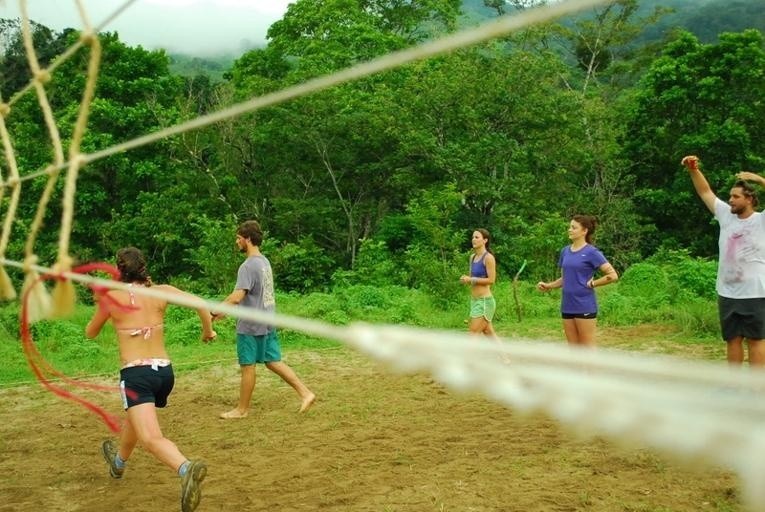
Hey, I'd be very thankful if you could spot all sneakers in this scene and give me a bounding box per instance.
[179,461,207,511]
[103,439,124,478]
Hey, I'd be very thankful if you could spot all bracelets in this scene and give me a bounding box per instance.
[472,277,478,284]
[210,312,218,317]
[590,277,595,288]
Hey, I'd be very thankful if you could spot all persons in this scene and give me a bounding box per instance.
[212,220,315,419]
[681,155,765,393]
[537,214,618,374]
[85,247,216,512]
[460,228,508,369]
